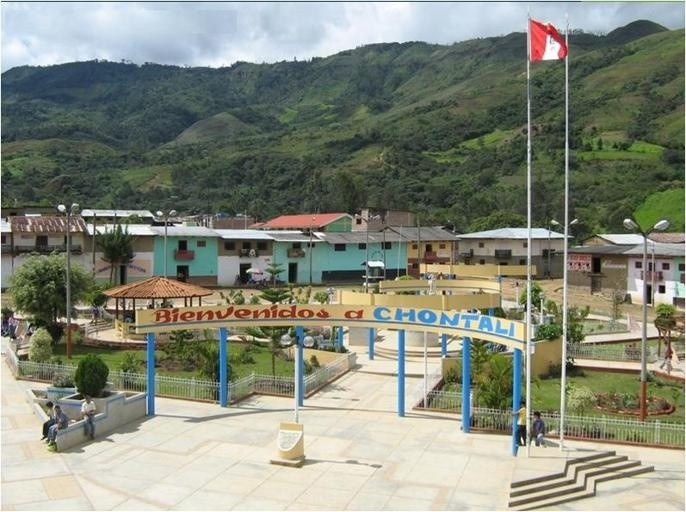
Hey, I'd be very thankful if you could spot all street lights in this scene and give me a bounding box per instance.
[355,211,385,293]
[551,217,578,287]
[57,200,81,359]
[308,215,317,286]
[623,217,671,422]
[155,208,179,278]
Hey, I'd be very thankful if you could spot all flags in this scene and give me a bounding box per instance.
[528,15,567,62]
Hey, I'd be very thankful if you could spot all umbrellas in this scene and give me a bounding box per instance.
[246,267,264,275]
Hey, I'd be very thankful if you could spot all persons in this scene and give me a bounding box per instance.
[41,401,55,440]
[26,323,33,336]
[8,314,15,338]
[89,305,98,326]
[533,411,545,447]
[81,396,96,440]
[511,401,526,445]
[45,406,68,445]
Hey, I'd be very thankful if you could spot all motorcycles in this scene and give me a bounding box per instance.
[254,277,270,290]
